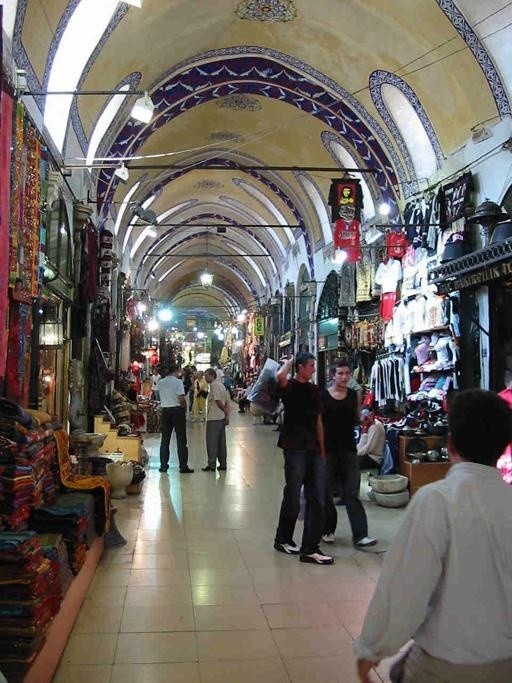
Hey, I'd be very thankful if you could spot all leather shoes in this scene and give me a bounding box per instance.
[217,466,226,470]
[202,465,215,472]
[180,467,194,472]
[159,467,167,472]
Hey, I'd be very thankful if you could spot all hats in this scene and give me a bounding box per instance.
[413,332,451,367]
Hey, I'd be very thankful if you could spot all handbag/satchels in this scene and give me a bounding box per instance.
[201,390,208,399]
[246,357,288,412]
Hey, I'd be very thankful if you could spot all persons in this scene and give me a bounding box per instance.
[151,364,252,472]
[274,352,387,565]
[354,388,512,682]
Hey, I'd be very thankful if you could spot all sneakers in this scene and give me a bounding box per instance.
[189,409,205,422]
[300,550,334,564]
[274,540,301,553]
[322,531,335,541]
[356,536,377,546]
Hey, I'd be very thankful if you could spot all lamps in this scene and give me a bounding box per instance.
[22,91,154,124]
[61,163,129,181]
[364,224,439,244]
[335,246,404,263]
[200,223,214,288]
